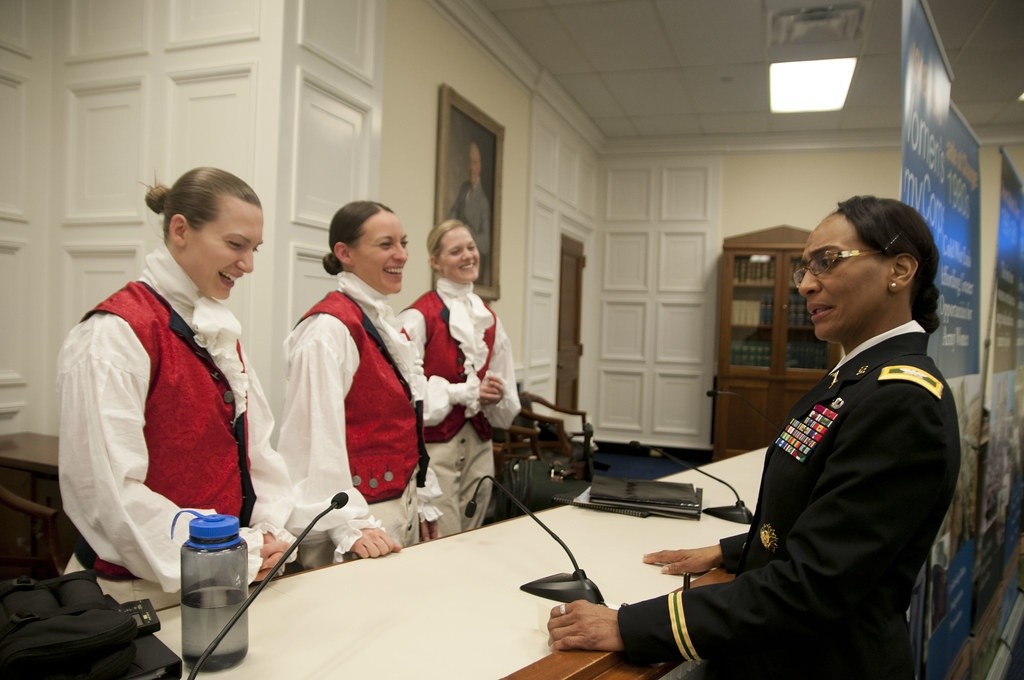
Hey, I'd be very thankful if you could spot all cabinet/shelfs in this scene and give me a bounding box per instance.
[708,225,842,458]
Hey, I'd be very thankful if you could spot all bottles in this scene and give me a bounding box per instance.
[171,511,248,673]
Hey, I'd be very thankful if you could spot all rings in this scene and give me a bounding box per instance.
[557,602,569,615]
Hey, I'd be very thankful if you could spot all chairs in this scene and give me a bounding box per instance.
[487,394,587,487]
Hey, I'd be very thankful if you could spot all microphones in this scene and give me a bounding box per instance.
[464,474,604,606]
[629,441,754,525]
[186,492,349,680]
[706,390,781,429]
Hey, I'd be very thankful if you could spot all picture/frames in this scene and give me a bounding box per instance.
[427,83,507,303]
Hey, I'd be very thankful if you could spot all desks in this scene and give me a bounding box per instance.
[152,447,770,680]
[0,433,58,570]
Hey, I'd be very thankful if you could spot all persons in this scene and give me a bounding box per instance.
[58,166,302,613]
[276,200,441,566]
[547,194,962,680]
[396,220,522,540]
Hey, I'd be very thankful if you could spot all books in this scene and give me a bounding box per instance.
[728,258,829,369]
[552,476,704,521]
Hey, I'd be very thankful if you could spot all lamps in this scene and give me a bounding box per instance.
[763,40,859,117]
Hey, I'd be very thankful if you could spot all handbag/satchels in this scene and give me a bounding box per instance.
[0,570,136,680]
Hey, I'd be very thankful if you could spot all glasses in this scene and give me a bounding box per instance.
[794,250,881,288]
